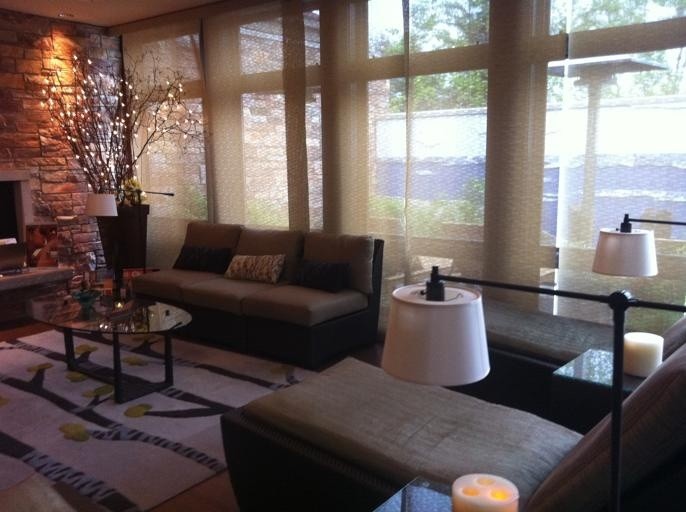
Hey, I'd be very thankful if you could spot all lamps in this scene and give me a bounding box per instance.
[87,194,118,216]
[381,266,686,512]
[592,214,686,277]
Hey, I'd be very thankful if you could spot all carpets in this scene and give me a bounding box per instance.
[0,319,319,512]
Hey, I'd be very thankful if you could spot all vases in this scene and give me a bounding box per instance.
[96,204,150,290]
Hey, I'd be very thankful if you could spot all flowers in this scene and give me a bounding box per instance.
[115,178,147,206]
[44,53,213,192]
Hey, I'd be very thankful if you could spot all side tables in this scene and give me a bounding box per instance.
[553,346,645,393]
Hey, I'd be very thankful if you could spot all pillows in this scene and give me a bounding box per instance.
[282,262,349,292]
[173,245,231,272]
[225,254,284,284]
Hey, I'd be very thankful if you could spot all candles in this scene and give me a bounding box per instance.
[453,472,520,512]
[622,331,663,379]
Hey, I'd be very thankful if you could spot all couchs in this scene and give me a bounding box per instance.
[131,219,384,369]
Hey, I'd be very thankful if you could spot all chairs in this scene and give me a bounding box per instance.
[222,341,686,512]
[482,298,686,373]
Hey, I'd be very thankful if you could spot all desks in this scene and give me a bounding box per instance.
[0,267,74,299]
